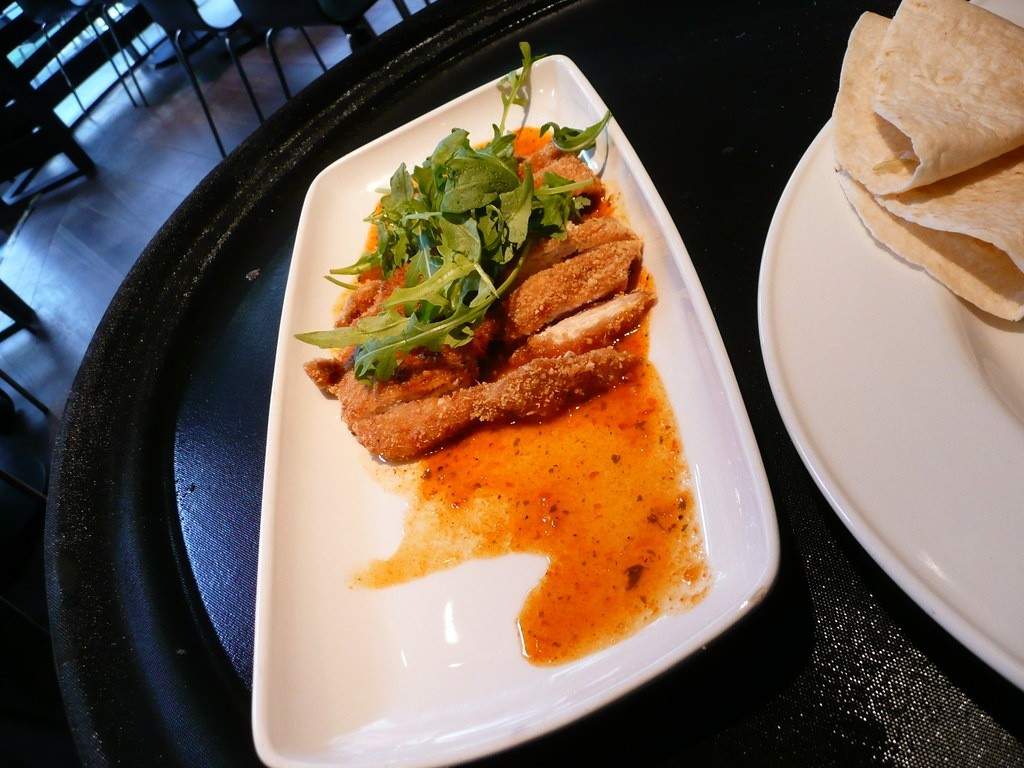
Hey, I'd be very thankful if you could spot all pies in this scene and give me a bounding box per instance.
[831,0,1024,323]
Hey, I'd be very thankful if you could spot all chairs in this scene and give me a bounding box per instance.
[0,0,435,243]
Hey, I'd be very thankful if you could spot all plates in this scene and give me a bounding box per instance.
[248,55,780,768]
[755,110,1024,689]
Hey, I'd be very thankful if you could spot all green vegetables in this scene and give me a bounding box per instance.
[291,42,612,388]
